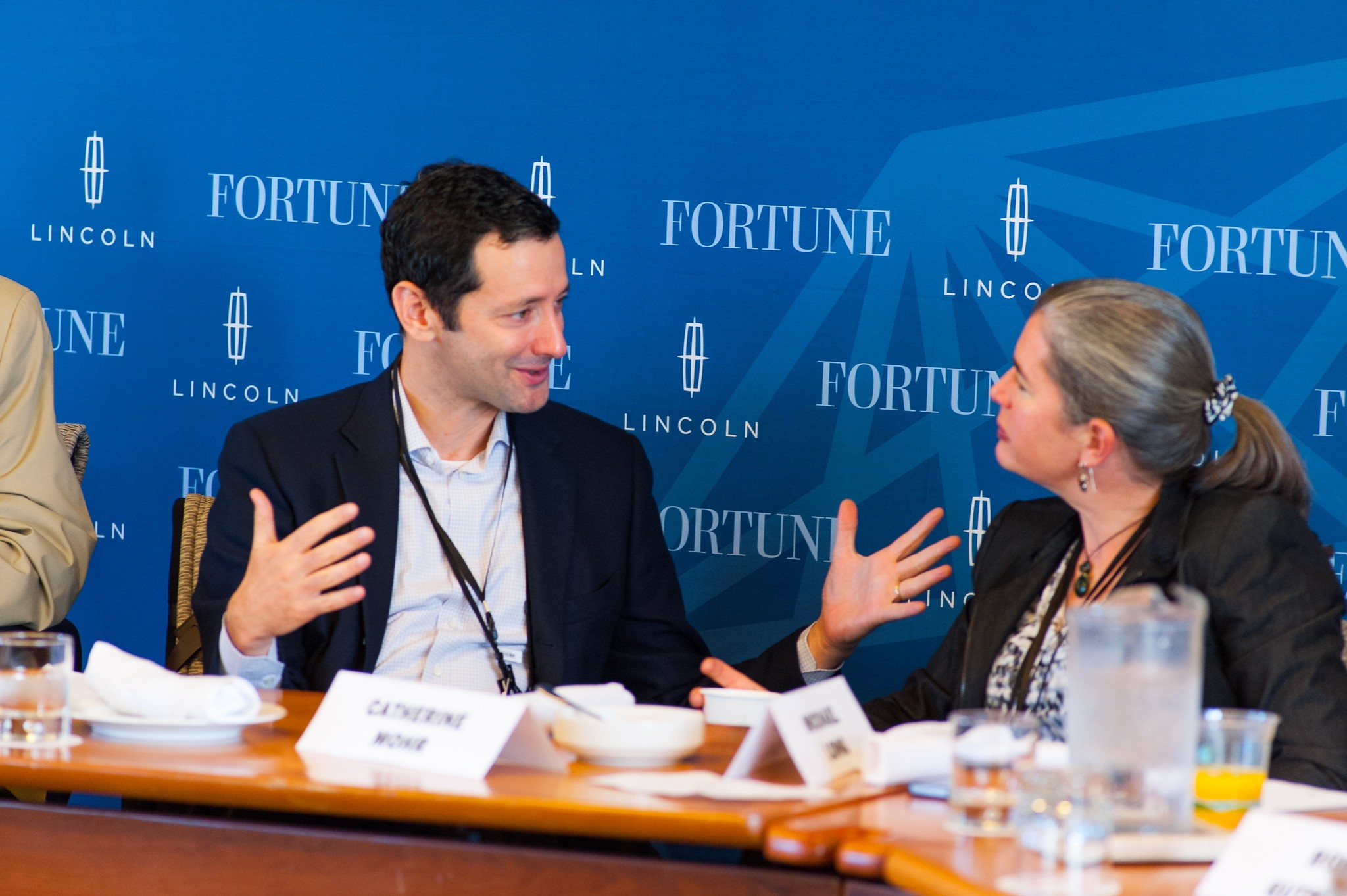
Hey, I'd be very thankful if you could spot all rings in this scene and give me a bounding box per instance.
[895,583,899,595]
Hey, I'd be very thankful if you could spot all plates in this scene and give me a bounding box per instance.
[550,704,706,766]
[71,704,290,747]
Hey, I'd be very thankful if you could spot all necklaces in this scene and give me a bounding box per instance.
[1075,516,1147,597]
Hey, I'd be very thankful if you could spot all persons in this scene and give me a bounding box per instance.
[0,275,96,632]
[190,158,960,709]
[690,279,1347,788]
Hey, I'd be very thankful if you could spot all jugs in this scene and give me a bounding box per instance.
[1065,595,1207,838]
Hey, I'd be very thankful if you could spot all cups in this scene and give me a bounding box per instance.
[1196,709,1283,831]
[0,632,75,752]
[948,708,1037,838]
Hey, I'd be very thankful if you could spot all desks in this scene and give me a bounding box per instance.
[1,668,1208,896]
